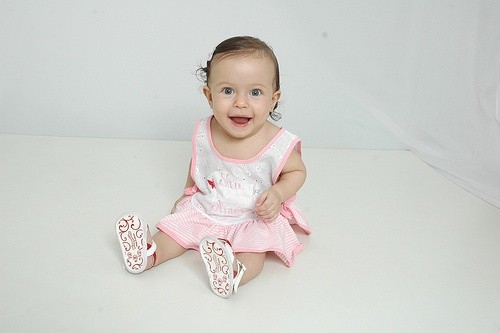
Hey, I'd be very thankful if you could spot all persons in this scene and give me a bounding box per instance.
[114,37,312,298]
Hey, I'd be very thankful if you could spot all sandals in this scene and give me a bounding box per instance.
[200,237,246,298]
[115,215,157,273]
